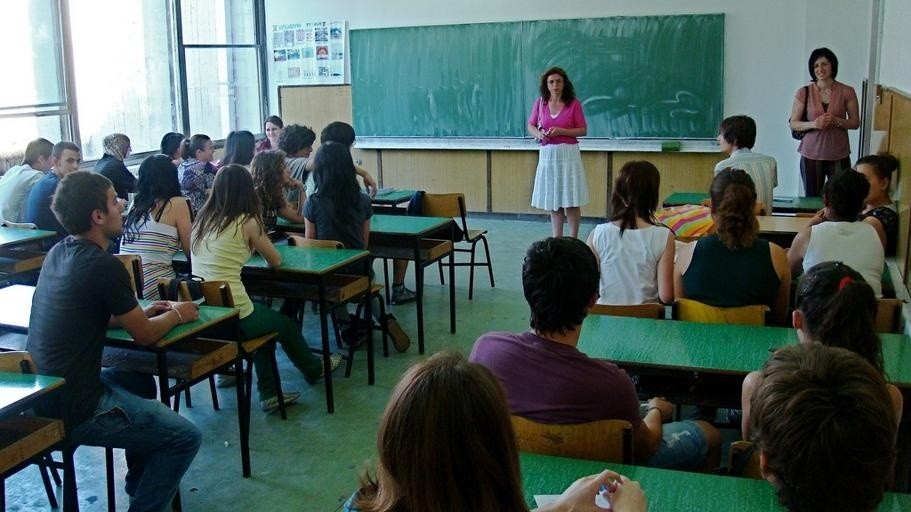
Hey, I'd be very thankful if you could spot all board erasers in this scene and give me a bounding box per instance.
[659,144,681,154]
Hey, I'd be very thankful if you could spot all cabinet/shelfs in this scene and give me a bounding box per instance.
[489,150,611,220]
[380,148,490,216]
[278,84,381,189]
[608,152,730,213]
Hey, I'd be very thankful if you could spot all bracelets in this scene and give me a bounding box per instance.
[835,116,845,128]
[645,406,667,418]
[170,305,184,327]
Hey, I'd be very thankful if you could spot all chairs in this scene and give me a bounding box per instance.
[701,198,765,214]
[672,298,769,325]
[413,191,495,300]
[874,298,904,334]
[2,350,110,512]
[158,276,288,442]
[2,220,39,230]
[289,234,389,377]
[112,254,144,302]
[588,302,665,320]
[507,412,634,467]
[735,441,777,481]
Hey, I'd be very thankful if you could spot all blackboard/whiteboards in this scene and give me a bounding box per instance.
[348,13,725,141]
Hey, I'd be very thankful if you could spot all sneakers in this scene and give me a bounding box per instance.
[380,313,410,353]
[391,288,417,305]
[305,353,342,383]
[216,366,247,388]
[341,324,369,347]
[261,391,301,411]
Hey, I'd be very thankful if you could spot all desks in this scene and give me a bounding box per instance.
[0,367,79,512]
[0,285,251,512]
[517,450,911,512]
[277,215,457,356]
[663,192,823,214]
[176,244,375,415]
[362,186,421,201]
[575,313,911,410]
[0,227,58,284]
[719,215,811,249]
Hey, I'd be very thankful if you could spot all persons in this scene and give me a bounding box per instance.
[188,164,342,413]
[160,132,188,174]
[220,131,253,171]
[178,135,220,216]
[248,150,304,234]
[584,160,676,402]
[751,342,896,512]
[528,67,586,237]
[116,153,245,387]
[305,143,411,352]
[24,170,203,511]
[24,144,121,253]
[728,263,904,497]
[305,122,420,306]
[258,116,285,153]
[0,138,53,228]
[464,236,722,472]
[715,116,779,215]
[791,48,860,195]
[780,175,886,322]
[676,167,790,426]
[277,125,319,208]
[846,156,900,257]
[344,352,649,511]
[94,136,140,201]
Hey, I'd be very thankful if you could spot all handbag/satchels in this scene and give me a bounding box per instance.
[535,96,544,144]
[788,86,809,141]
